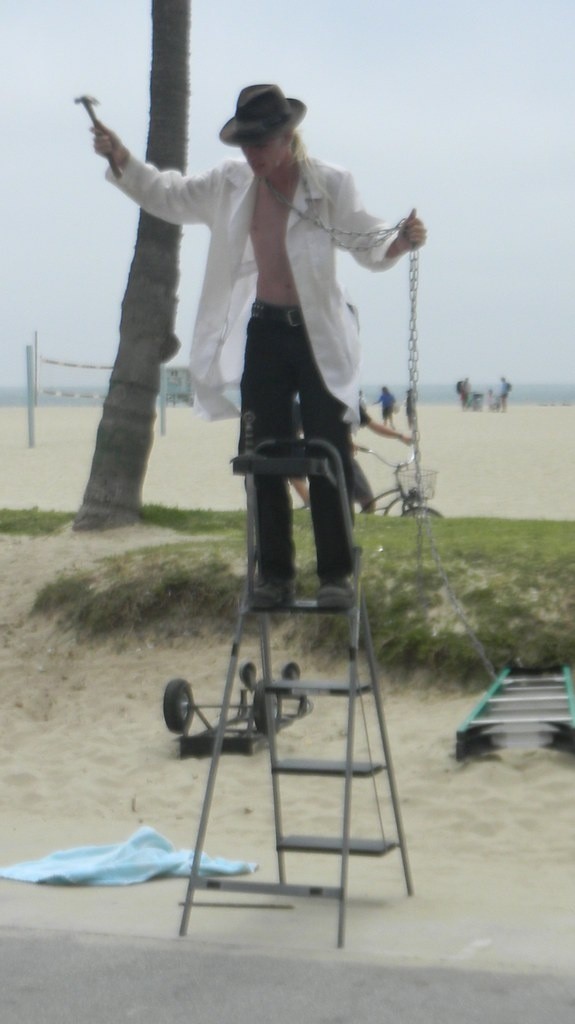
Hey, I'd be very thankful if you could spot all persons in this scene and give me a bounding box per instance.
[91,84,426,610]
[282,378,512,516]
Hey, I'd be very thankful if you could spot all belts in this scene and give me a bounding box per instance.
[251,303,302,326]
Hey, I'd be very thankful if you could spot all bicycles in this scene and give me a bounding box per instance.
[289,441,448,524]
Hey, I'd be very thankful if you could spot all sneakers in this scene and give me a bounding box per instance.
[249,579,296,608]
[318,575,354,607]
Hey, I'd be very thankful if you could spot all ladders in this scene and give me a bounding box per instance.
[178,434,417,948]
[451,664,575,766]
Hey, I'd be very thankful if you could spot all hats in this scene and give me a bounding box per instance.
[219,84,307,147]
